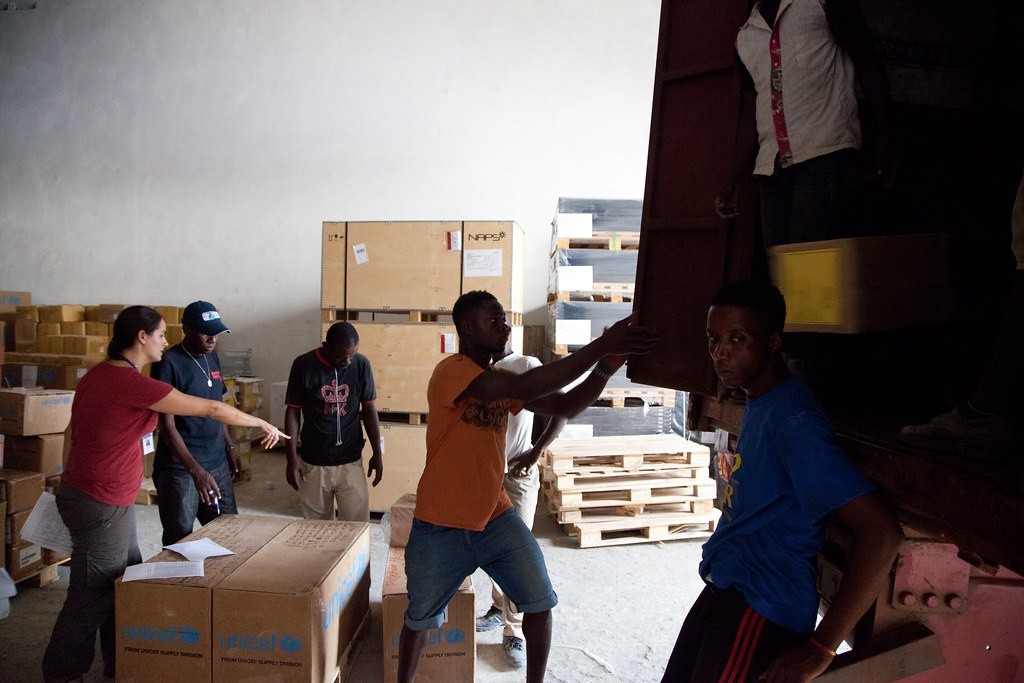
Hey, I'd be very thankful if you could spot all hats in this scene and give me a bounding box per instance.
[181,300,231,337]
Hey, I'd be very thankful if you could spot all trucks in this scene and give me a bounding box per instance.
[626,0,1024,683]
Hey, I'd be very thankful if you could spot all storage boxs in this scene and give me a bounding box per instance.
[766,234,953,336]
[114,513,371,683]
[382,493,477,683]
[0,290,264,580]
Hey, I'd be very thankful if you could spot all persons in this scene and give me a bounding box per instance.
[396,291,662,683]
[714,0,897,249]
[151,302,238,550]
[898,177,1024,462]
[284,321,384,521]
[476,316,567,668]
[662,275,904,683]
[42,305,291,683]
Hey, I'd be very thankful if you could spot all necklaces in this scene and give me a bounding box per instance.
[182,342,212,387]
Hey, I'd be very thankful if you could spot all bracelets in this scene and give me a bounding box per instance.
[594,368,610,380]
[809,636,836,655]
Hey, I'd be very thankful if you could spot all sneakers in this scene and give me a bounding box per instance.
[476,606,505,632]
[503,635,525,667]
